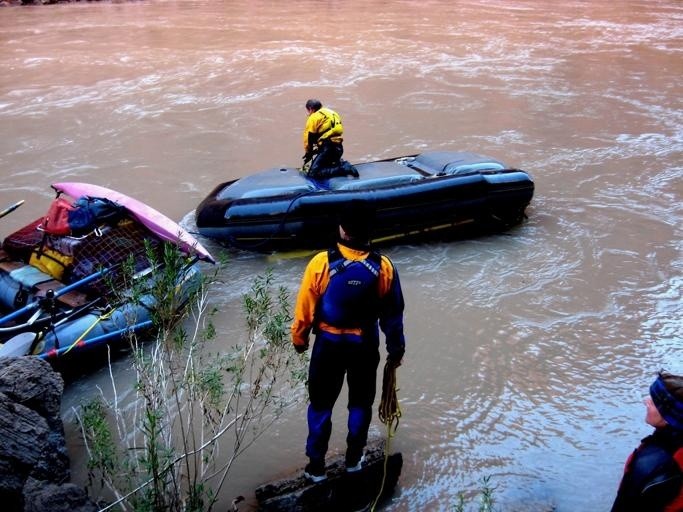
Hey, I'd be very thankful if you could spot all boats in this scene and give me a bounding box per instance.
[194,151,534,253]
[1,182,204,375]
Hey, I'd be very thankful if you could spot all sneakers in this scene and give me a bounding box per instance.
[345,456,363,473]
[301,465,329,484]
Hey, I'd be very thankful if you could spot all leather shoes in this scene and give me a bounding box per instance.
[341,161,360,179]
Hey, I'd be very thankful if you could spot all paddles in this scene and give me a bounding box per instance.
[0,263,163,356]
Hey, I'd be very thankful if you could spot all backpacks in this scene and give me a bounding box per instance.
[37,196,76,258]
[313,246,382,331]
[68,195,122,234]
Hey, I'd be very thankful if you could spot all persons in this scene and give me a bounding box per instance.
[303,99,360,178]
[291,202,406,481]
[611,374,683,512]
